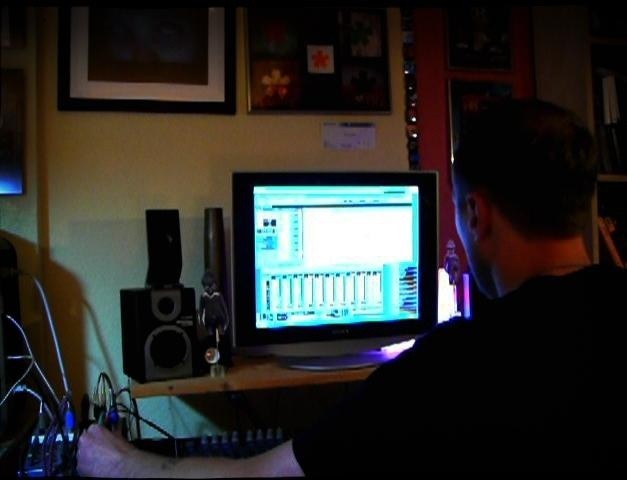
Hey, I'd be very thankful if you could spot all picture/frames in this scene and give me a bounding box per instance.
[443,0,515,74]
[242,0,393,116]
[447,78,514,187]
[58,0,237,114]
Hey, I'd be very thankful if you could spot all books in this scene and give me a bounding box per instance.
[594,75,627,174]
[599,214,625,271]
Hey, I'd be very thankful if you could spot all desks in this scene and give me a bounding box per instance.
[129,353,375,458]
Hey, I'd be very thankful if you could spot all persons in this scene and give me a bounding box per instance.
[76,95,627,480]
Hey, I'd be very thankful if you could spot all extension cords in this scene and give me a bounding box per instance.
[31,421,125,444]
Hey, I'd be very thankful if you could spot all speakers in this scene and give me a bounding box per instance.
[144,209,184,290]
[119,286,202,383]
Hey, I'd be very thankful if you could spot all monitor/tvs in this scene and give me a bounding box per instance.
[230,170,440,370]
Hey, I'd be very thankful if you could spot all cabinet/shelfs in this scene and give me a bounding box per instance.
[533,0,627,265]
